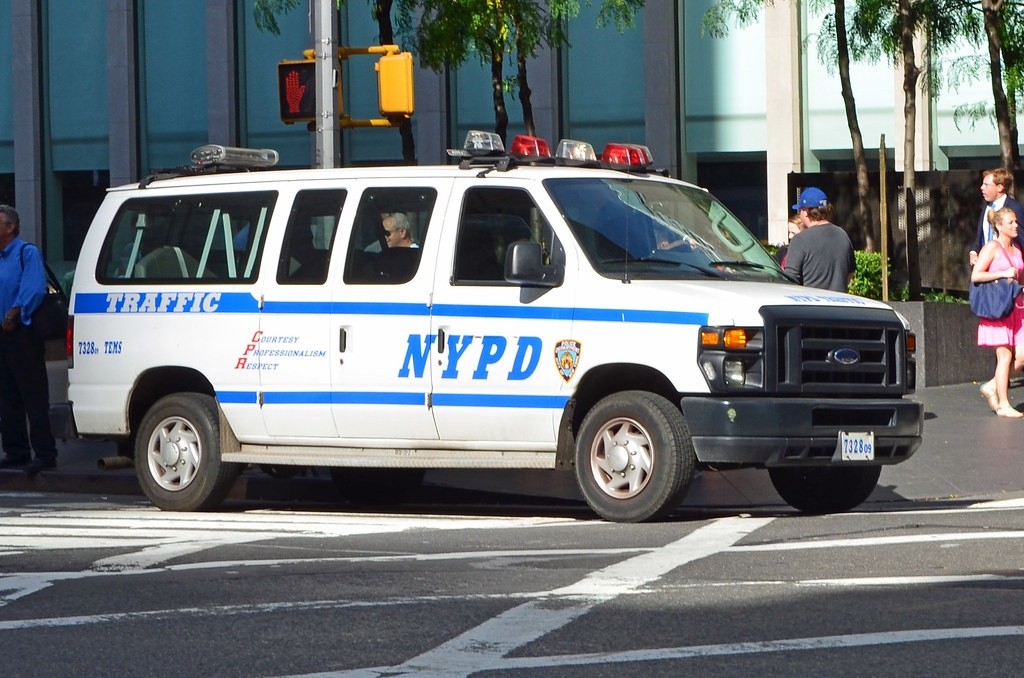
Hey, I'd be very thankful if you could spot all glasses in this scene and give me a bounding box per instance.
[385,229,403,236]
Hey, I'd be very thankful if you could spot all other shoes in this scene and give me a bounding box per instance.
[980,383,998,411]
[0,453,31,468]
[23,454,57,472]
[996,405,1023,418]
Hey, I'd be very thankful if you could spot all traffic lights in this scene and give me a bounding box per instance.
[278,60,316,123]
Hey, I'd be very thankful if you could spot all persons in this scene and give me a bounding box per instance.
[774,187,858,294]
[969,168,1024,276]
[0,204,57,474]
[381,213,419,248]
[970,208,1024,419]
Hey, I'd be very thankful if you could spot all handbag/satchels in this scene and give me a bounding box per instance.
[20,243,67,342]
[968,239,1022,318]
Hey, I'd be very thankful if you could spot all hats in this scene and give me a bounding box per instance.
[792,187,827,209]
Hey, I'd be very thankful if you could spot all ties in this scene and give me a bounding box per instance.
[988,203,996,242]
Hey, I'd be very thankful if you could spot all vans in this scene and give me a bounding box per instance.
[63,132,926,525]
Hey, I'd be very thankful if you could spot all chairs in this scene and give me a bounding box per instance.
[135,246,217,278]
[349,247,421,283]
[481,224,521,263]
